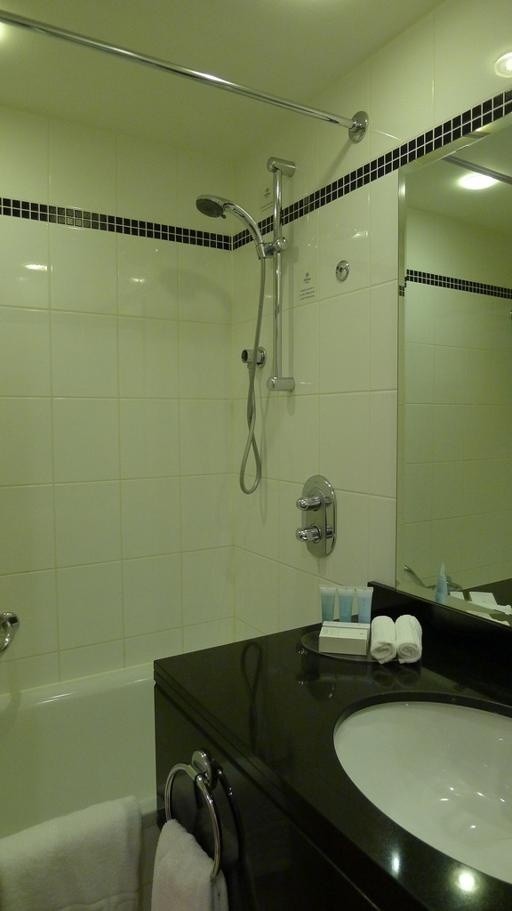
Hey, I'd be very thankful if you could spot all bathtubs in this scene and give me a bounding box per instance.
[0,662,160,911]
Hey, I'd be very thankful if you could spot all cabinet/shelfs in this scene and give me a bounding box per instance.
[154,684,380,911]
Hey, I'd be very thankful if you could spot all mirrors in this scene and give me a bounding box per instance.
[393,113,512,629]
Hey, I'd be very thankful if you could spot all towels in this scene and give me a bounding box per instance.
[151,819,228,911]
[0,794,144,911]
[394,614,422,664]
[370,615,396,662]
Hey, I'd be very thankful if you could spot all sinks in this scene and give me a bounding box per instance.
[328,687,512,888]
